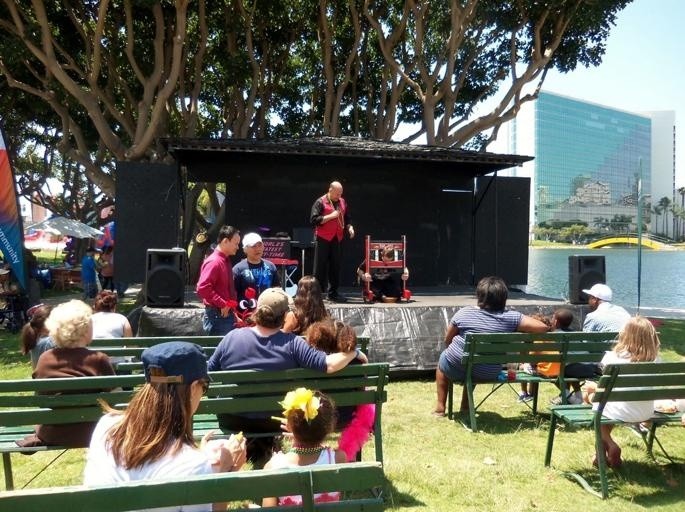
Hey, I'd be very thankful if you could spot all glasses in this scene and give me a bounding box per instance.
[200,383,210,396]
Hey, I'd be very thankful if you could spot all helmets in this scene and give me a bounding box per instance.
[141,341,212,385]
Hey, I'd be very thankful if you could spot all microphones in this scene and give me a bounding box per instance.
[335,201,339,210]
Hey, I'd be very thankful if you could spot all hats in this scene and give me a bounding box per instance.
[241,232,262,248]
[581,282,612,302]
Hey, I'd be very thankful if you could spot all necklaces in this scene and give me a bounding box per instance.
[327,197,345,230]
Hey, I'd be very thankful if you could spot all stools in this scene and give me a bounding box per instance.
[263,256,299,291]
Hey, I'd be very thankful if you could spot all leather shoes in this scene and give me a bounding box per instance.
[326,293,346,303]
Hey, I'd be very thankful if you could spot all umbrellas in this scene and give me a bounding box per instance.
[22,213,104,263]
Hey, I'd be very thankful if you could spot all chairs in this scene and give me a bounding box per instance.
[49,266,84,296]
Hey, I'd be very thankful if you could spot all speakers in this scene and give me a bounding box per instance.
[145,248,186,308]
[568,255,605,304]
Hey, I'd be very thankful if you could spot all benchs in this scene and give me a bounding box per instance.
[444,331,662,435]
[0,453,393,512]
[26,335,375,394]
[1,359,393,511]
[543,361,685,503]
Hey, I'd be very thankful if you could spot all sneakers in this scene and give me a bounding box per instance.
[518,390,529,403]
[525,393,533,403]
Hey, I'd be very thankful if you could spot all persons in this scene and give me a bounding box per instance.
[593,315,664,466]
[0,243,133,456]
[551,284,632,404]
[309,181,355,301]
[86,225,378,512]
[550,309,596,405]
[517,313,561,402]
[431,276,550,417]
[357,248,410,302]
